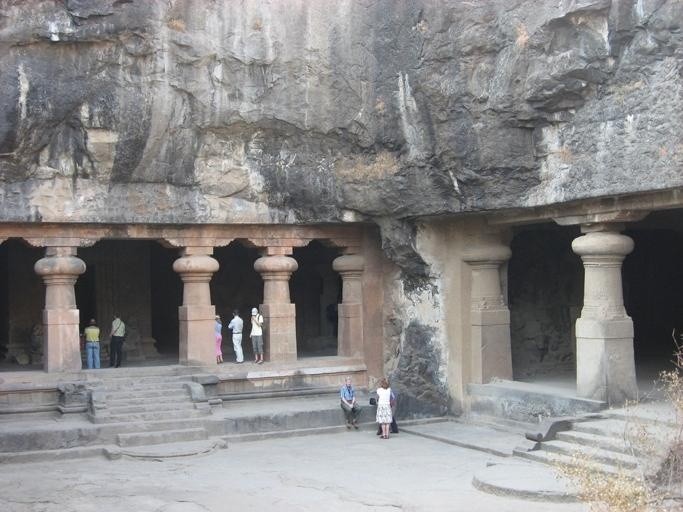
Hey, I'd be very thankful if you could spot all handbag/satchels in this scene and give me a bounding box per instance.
[390,400,397,406]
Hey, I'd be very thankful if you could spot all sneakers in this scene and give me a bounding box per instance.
[345,420,358,428]
[253,359,264,364]
[377,428,398,439]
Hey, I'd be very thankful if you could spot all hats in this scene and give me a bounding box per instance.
[252,308,258,316]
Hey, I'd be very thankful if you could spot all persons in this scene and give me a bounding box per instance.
[108,313,125,366]
[248,307,266,365]
[375,379,394,439]
[227,308,244,364]
[81,318,101,369]
[213,315,225,364]
[376,380,398,436]
[338,376,362,428]
